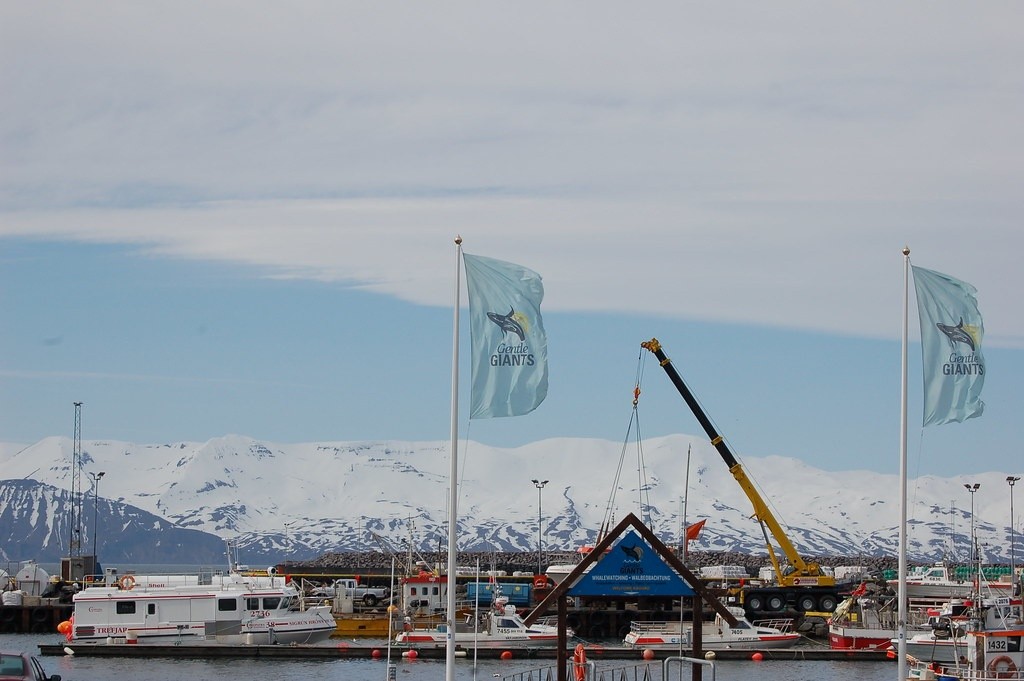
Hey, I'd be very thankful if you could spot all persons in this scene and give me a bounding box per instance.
[959,656,968,664]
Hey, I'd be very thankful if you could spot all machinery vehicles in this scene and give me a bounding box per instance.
[595,338,837,612]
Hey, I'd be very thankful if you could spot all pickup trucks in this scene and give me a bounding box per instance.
[310,579,388,606]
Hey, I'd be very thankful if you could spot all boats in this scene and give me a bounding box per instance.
[890,599,1024,662]
[72,567,338,645]
[886,567,1012,598]
[546,547,610,588]
[828,582,962,649]
[906,630,1024,681]
[392,596,575,648]
[624,607,801,649]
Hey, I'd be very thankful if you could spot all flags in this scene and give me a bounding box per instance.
[911,266,987,427]
[462,252,548,419]
[685,520,707,548]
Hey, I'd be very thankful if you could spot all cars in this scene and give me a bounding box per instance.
[0,651,61,681]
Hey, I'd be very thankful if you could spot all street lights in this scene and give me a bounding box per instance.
[284,524,289,573]
[357,517,365,568]
[89,472,105,587]
[1006,476,1021,598]
[531,479,549,575]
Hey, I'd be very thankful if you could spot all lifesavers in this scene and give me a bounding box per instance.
[990,654,1017,678]
[119,575,135,589]
[573,643,585,680]
